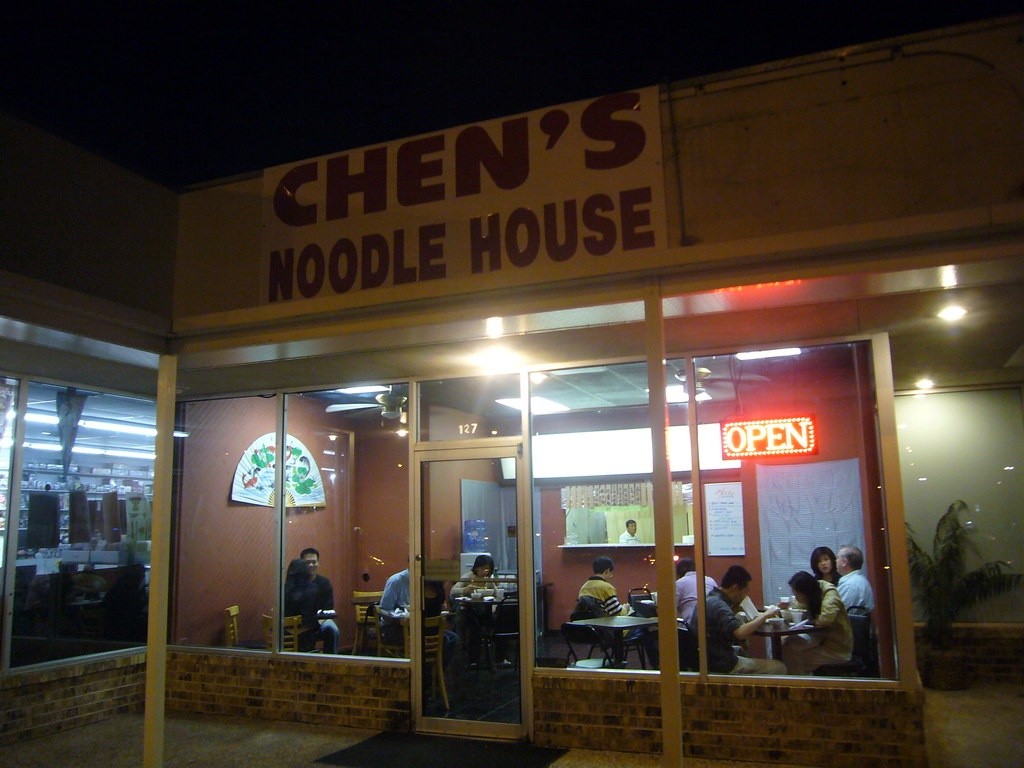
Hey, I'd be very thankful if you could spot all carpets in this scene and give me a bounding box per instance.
[310,730,571,768]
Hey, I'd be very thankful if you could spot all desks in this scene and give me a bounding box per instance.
[455,597,519,671]
[560,616,659,670]
[741,621,827,663]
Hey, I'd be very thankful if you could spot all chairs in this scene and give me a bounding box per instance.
[78,607,104,641]
[222,591,878,709]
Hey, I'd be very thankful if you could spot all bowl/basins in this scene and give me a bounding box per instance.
[739,613,763,623]
[477,589,494,596]
[780,609,807,621]
[471,593,482,598]
[769,619,785,627]
[402,605,410,611]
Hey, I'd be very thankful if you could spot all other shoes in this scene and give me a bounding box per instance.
[494,658,511,666]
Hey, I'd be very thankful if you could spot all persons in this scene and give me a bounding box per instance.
[450,555,512,667]
[577,556,659,670]
[284,548,339,655]
[619,520,640,545]
[675,557,788,674]
[778,544,875,676]
[378,557,445,658]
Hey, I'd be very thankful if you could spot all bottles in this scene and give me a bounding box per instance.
[464,519,489,553]
[572,522,579,545]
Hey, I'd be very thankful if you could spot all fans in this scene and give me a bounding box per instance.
[624,353,769,397]
[324,384,409,421]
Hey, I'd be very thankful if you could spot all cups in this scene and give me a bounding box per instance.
[791,613,803,624]
[495,589,505,602]
[780,597,790,609]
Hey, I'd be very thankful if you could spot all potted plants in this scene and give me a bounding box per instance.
[904,505,1024,693]
